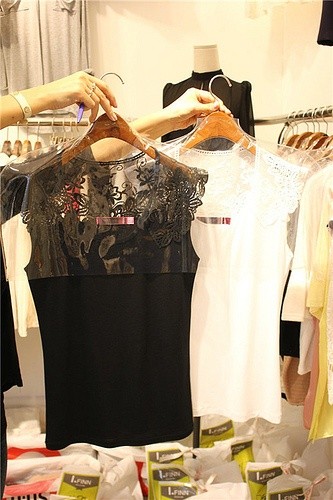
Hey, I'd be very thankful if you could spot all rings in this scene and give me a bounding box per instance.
[88,89,93,96]
[92,83,96,90]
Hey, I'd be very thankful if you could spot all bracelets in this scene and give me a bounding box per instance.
[10,91,31,118]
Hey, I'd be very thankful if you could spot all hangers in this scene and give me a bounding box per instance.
[0,120,80,159]
[62,72,194,180]
[179,75,256,156]
[276,105,333,178]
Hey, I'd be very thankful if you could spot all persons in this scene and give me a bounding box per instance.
[163,45,256,161]
[0,72,234,500]
